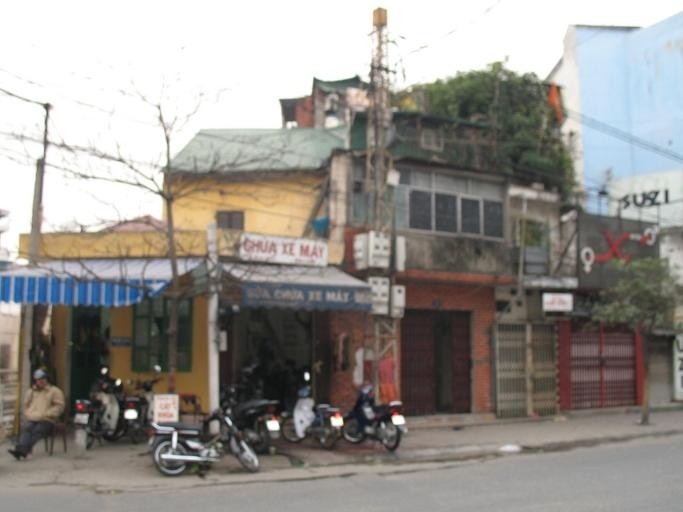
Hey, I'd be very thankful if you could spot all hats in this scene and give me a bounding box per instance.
[33,369,47,380]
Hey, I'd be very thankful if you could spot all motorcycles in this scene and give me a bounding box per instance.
[281,364,342,452]
[138,396,261,474]
[343,383,409,453]
[229,363,282,453]
[64,362,165,450]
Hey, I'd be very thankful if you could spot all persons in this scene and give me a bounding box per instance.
[8,368,66,461]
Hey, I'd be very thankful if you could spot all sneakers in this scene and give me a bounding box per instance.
[8,445,27,460]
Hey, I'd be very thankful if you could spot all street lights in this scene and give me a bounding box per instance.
[370,5,389,402]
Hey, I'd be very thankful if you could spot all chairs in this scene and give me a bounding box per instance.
[48,397,69,454]
